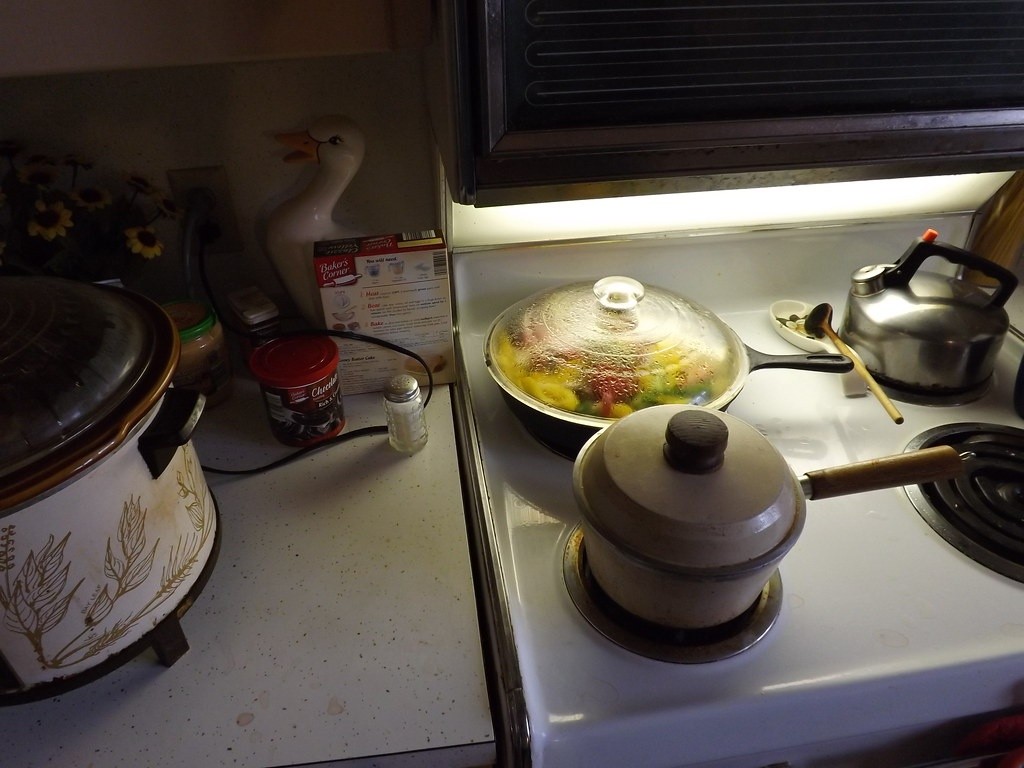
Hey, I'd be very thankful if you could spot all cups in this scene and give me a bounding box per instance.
[158,299,227,408]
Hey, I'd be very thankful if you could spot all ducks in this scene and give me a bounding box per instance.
[262,117,370,327]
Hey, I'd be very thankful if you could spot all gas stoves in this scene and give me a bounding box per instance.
[439,5,1024,667]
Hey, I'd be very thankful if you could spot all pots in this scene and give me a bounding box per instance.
[834,228,1018,393]
[0,272,223,706]
[483,275,856,458]
[571,403,960,630]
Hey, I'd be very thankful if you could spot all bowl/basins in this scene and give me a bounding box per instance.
[768,299,868,396]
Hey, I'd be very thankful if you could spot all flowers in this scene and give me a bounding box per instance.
[0,141,185,262]
